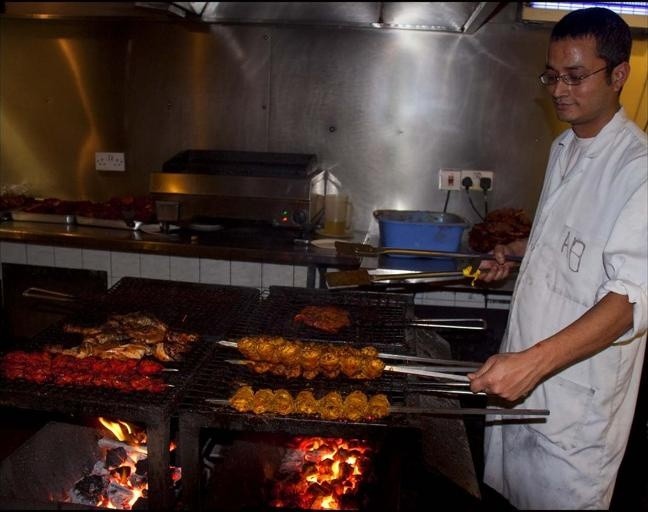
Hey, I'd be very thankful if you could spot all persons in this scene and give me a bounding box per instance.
[465,7,646,512]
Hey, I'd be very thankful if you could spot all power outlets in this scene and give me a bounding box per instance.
[95,153,125,171]
[460,170,493,193]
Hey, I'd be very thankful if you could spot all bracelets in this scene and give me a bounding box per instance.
[505,245,516,267]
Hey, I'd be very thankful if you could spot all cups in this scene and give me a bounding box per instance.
[324,195,354,234]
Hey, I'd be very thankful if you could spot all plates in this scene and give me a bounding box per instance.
[315,228,356,239]
[140,221,179,235]
[310,239,352,249]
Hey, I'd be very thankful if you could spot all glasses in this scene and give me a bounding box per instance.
[538,66,607,86]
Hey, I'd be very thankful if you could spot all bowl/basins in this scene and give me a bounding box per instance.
[373,209,472,260]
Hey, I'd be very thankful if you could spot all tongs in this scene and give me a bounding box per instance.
[324,241,524,290]
